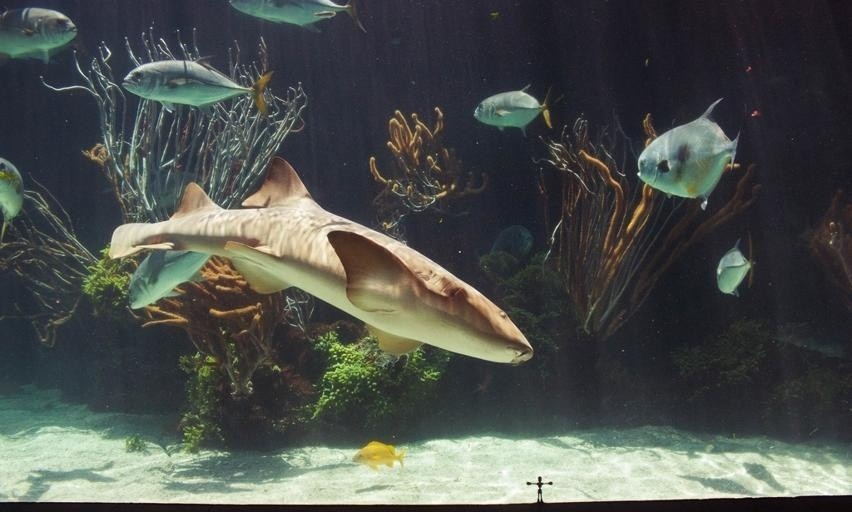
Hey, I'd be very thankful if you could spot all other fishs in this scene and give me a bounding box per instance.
[633,94,742,213]
